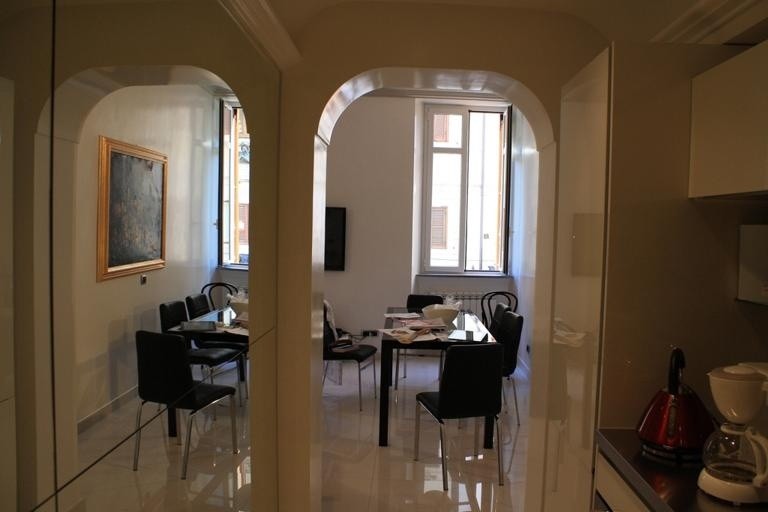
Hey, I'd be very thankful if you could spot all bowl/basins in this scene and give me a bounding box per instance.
[230,300,249,317]
[422,303,458,326]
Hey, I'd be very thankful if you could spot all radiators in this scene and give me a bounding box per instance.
[427,291,496,328]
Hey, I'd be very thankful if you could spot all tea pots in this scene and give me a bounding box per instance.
[637,348,720,469]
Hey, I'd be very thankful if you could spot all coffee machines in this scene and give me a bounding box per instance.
[695,364,768,508]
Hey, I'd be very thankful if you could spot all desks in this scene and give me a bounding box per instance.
[378,307,499,449]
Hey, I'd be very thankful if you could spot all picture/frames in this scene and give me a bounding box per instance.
[98,136,171,286]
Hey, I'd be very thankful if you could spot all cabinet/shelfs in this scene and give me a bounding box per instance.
[688,41,768,199]
[590,448,648,512]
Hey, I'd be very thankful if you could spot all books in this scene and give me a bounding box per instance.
[405,317,447,330]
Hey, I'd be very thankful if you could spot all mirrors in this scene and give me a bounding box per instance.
[52,8,281,508]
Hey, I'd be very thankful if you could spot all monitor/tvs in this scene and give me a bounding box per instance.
[324,206,346,270]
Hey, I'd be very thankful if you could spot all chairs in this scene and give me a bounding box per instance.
[412,342,504,491]
[408,295,443,312]
[489,302,509,333]
[493,312,523,425]
[481,292,518,327]
[131,280,252,481]
[324,300,376,410]
